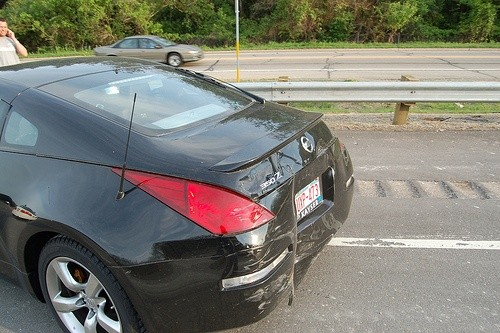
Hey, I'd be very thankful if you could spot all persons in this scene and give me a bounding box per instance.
[0,18,28,68]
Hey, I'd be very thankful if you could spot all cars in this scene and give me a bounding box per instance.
[0,57,355,332]
[93,35,204,67]
